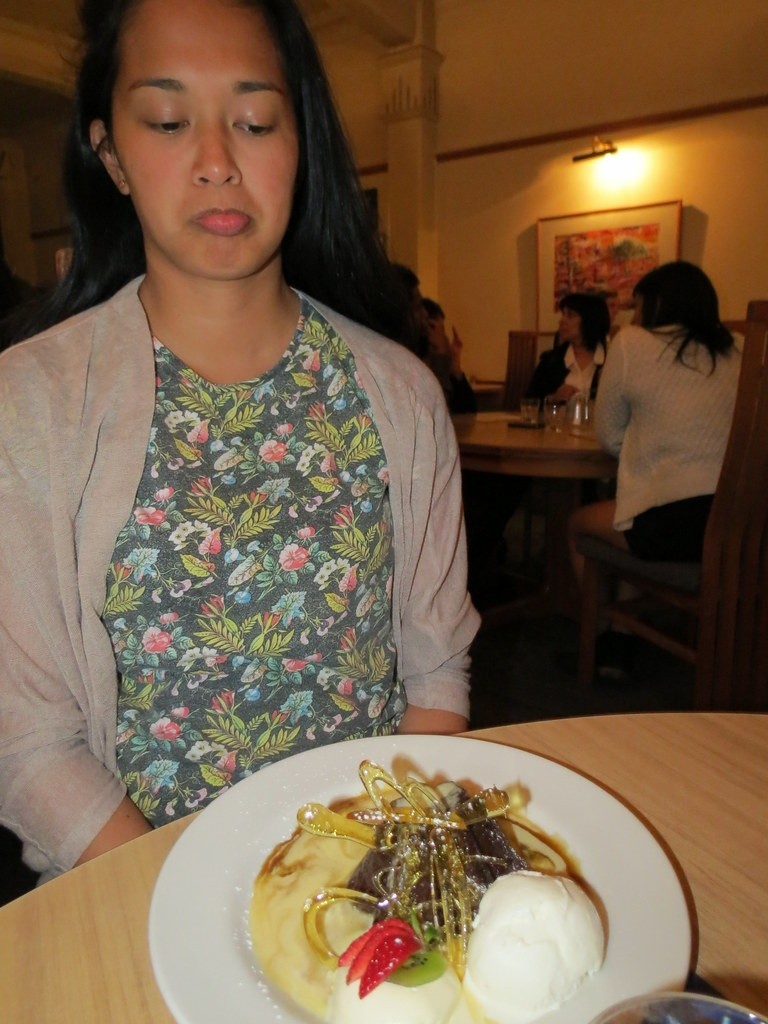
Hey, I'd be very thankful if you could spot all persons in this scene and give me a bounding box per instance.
[1,0,480,908]
[411,260,751,679]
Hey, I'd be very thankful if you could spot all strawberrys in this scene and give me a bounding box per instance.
[337,918,421,999]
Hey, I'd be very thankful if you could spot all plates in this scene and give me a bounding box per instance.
[148,735,692,1023]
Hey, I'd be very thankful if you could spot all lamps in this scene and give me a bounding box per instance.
[573,135,616,162]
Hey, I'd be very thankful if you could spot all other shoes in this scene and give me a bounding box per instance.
[562,647,629,682]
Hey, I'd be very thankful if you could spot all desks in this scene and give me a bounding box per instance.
[451,410,616,621]
[0,715,768,1024]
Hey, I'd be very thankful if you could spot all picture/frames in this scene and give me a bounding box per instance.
[536,199,683,337]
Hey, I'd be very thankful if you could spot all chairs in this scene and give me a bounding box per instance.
[502,330,538,412]
[577,301,768,715]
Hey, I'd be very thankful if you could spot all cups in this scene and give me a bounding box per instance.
[566,395,588,425]
[588,992,767,1024]
[544,395,567,432]
[520,397,540,425]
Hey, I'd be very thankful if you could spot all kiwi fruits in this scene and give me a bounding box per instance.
[384,949,446,986]
